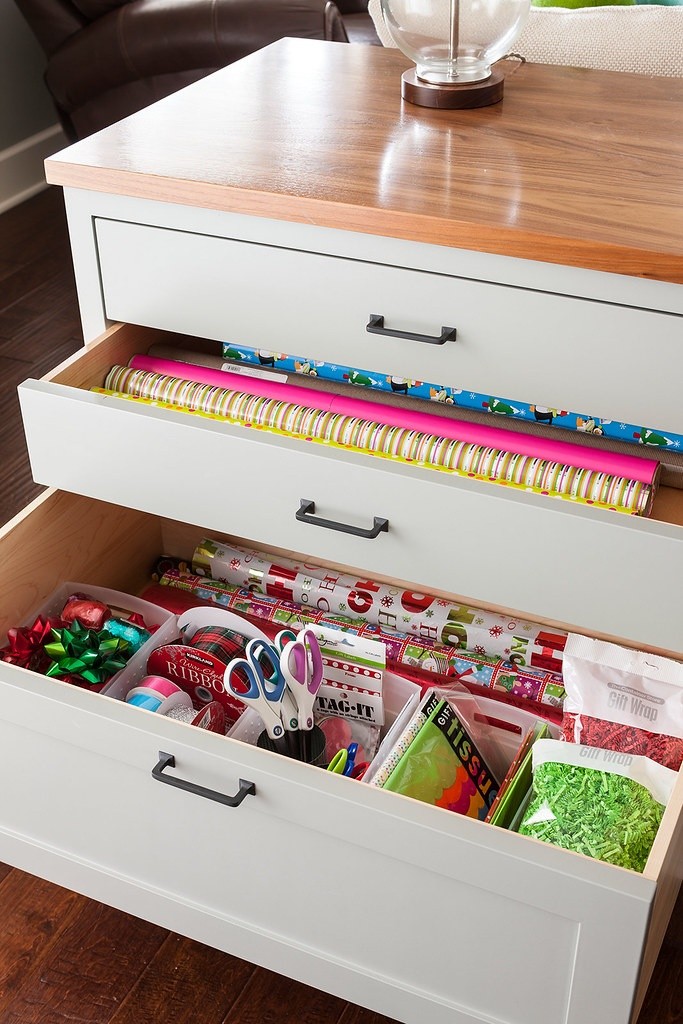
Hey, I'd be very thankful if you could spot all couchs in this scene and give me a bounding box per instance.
[16,0,385,143]
[368,0,683,77]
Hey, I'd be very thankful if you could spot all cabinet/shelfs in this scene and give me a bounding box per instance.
[0,36,683,1024]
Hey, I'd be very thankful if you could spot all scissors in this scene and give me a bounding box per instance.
[223,631,320,756]
[327,742,369,781]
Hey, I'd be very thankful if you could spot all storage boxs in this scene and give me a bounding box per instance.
[0,582,564,832]
